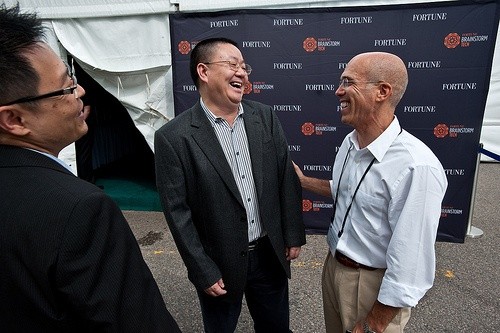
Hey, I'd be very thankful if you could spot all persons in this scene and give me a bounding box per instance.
[291,52,448,333]
[154,37,306,333]
[0,0,183,333]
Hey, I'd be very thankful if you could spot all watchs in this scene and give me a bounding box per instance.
[361,319,376,333]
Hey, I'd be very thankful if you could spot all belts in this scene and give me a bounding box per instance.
[247,240,269,253]
[334,249,375,270]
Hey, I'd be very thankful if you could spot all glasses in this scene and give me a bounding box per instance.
[1,60,79,106]
[339,78,382,88]
[202,61,252,76]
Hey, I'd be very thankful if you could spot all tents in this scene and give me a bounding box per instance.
[0,0,500,244]
